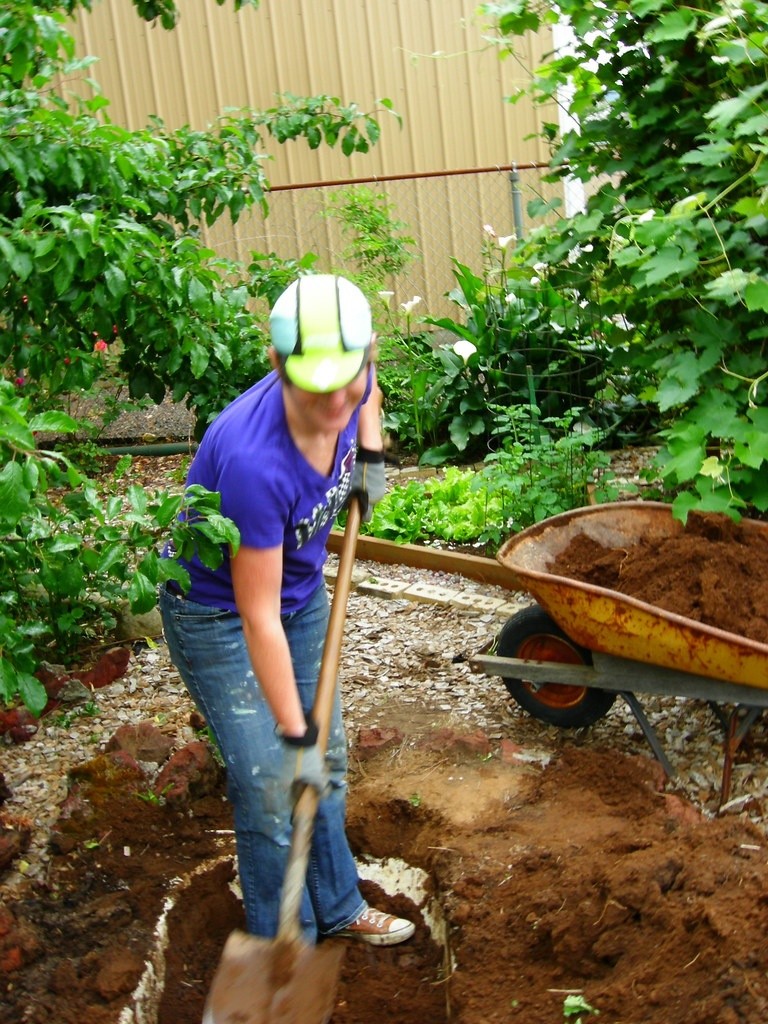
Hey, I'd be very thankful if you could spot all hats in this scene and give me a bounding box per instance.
[269,274,372,393]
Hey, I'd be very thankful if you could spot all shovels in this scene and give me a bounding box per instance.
[200,497,361,1024]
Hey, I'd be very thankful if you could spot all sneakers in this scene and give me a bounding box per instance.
[328,907,416,945]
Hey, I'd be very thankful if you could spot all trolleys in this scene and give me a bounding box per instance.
[473,500,768,813]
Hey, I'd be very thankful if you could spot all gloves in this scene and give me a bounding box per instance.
[274,716,346,825]
[341,448,385,521]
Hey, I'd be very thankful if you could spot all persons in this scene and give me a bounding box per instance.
[158,273,417,947]
